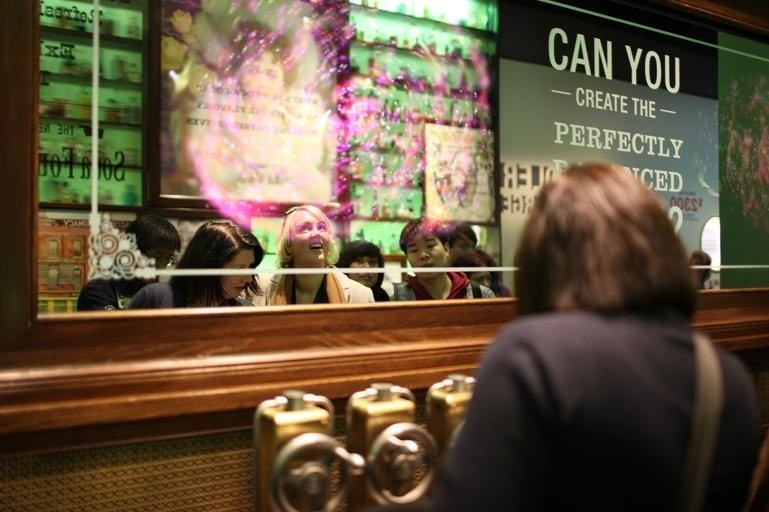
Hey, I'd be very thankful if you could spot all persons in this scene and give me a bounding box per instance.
[445,222,511,297]
[76,213,181,310]
[392,215,496,298]
[335,240,392,302]
[193,22,334,198]
[260,202,375,307]
[128,217,264,309]
[428,159,765,511]
[690,251,712,289]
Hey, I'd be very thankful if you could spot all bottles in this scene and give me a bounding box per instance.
[351,0,497,219]
[41,7,144,206]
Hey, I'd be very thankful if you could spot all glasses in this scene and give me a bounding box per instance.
[144,251,175,267]
